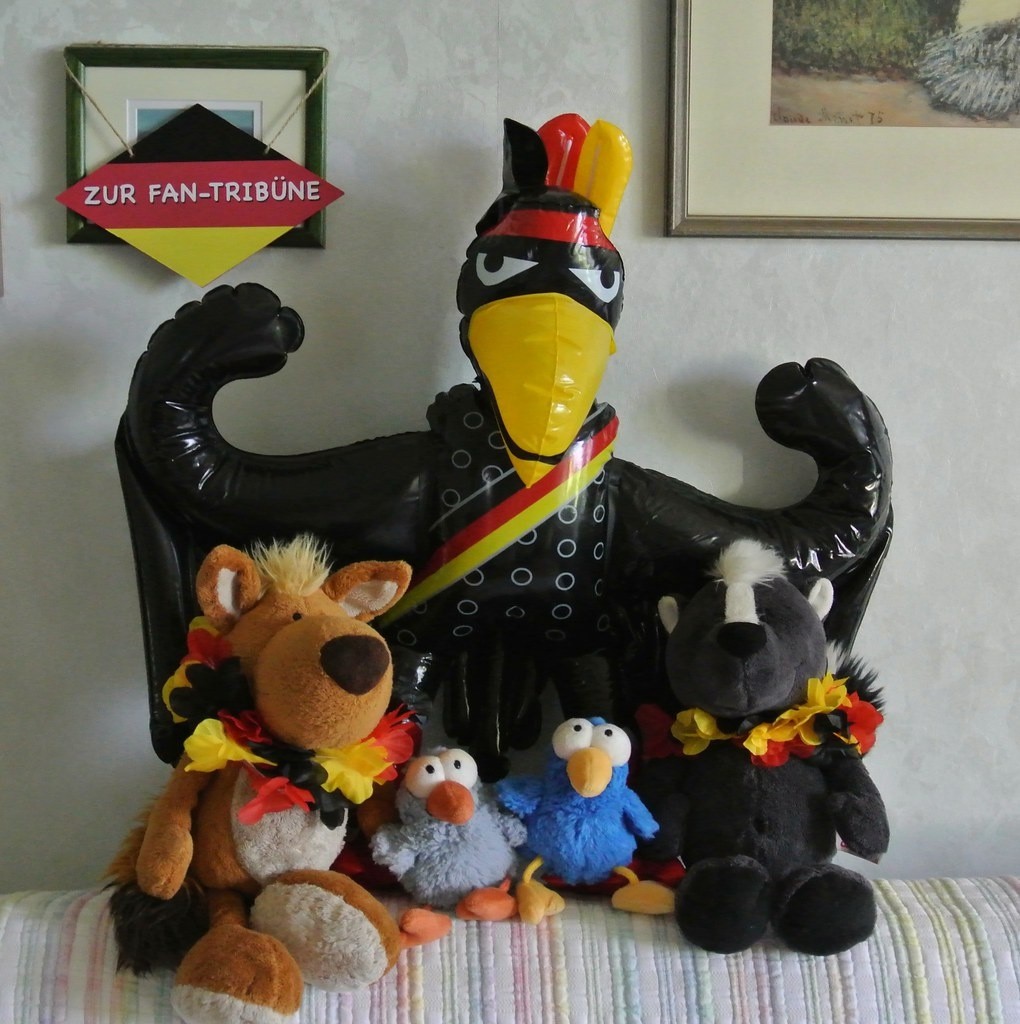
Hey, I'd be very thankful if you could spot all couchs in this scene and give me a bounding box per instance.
[0,875,1020,1024]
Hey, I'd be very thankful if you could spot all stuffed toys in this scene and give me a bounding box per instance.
[368,746,527,951]
[99,533,412,1024]
[492,716,676,925]
[638,537,890,957]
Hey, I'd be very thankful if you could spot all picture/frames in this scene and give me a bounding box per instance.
[664,0,1020,243]
[63,43,327,248]
